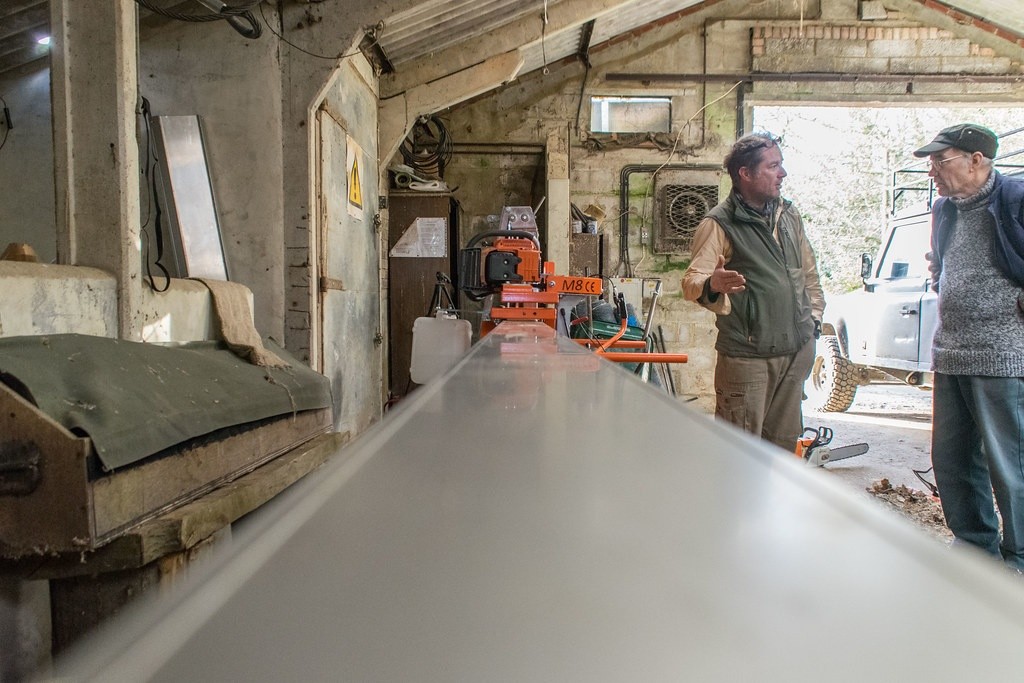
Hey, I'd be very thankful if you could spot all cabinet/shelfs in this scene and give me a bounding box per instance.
[388,192,464,408]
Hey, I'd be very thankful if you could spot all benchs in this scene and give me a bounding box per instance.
[8,430,353,660]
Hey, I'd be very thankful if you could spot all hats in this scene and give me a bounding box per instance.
[912,122,999,159]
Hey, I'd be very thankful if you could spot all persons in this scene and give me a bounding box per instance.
[913,123,1024,580]
[681,133,827,453]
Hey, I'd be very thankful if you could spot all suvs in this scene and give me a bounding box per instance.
[803,191,942,412]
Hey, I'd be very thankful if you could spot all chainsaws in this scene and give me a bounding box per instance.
[795,425,870,468]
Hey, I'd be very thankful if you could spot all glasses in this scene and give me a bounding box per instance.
[926,153,965,172]
[740,136,782,155]
